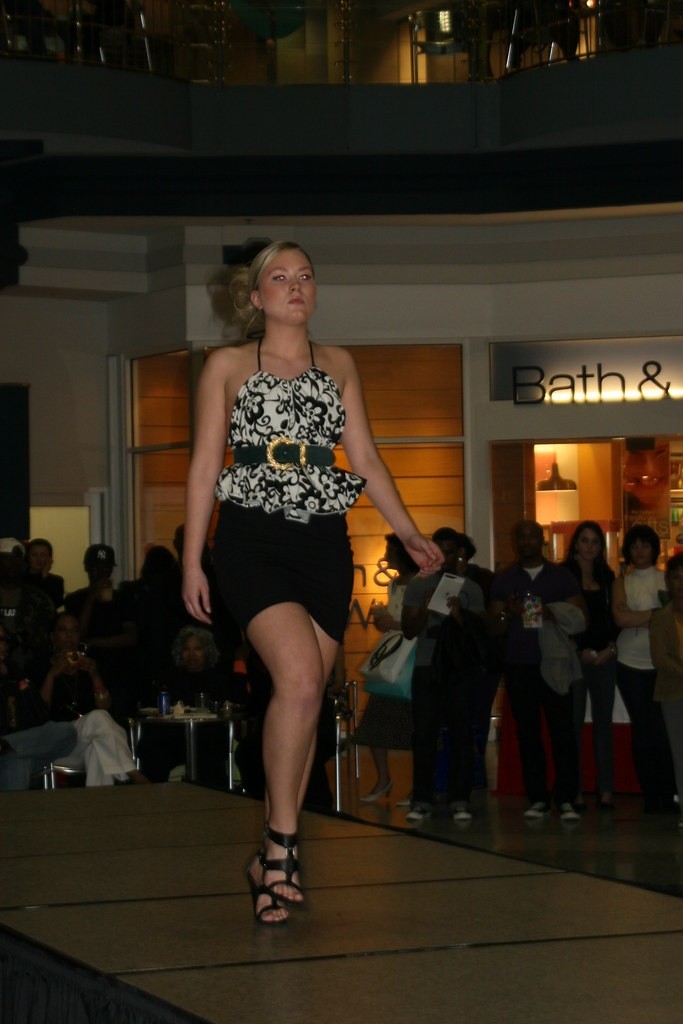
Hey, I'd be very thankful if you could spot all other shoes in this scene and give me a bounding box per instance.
[574,799,588,816]
[595,795,615,811]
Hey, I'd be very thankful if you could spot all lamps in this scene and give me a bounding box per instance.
[534,455,577,494]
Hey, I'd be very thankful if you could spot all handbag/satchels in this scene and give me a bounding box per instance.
[362,643,420,702]
[358,629,418,683]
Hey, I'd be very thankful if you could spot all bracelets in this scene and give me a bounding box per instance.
[608,646,617,654]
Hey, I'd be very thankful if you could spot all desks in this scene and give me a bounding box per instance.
[135,705,248,793]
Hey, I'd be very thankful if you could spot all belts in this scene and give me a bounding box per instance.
[231,437,335,471]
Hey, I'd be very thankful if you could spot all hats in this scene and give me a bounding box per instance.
[0,537,26,558]
[85,543,115,567]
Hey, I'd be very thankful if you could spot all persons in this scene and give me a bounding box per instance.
[0,0,135,69]
[354,519,683,822]
[0,523,346,818]
[183,235,447,925]
[504,0,669,73]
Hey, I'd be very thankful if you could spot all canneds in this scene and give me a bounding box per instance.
[157,691,169,717]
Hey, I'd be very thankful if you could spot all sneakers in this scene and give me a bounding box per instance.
[450,800,473,819]
[557,802,582,821]
[406,800,433,819]
[523,801,552,817]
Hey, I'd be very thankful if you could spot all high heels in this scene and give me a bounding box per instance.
[396,788,413,805]
[359,777,394,802]
[246,848,291,925]
[259,821,306,906]
